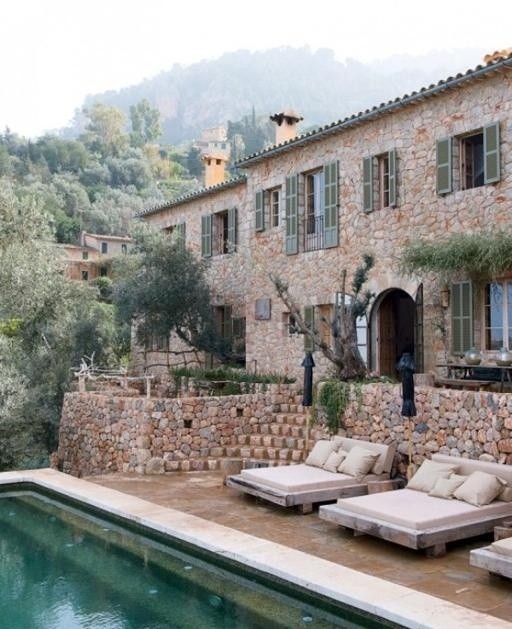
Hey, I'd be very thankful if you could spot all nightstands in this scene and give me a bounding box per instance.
[493,520,512,542]
[366,479,402,494]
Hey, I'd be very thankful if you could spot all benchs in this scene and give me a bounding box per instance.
[438,377,494,391]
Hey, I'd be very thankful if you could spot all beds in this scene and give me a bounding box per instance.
[225,434,397,514]
[468,536,512,581]
[317,453,512,558]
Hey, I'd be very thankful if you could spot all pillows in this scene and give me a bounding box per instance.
[451,470,506,508]
[322,452,345,473]
[305,439,341,469]
[371,462,383,476]
[403,458,459,493]
[499,488,512,502]
[337,446,380,481]
[449,472,466,482]
[427,478,463,501]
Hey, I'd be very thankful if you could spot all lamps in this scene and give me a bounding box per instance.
[440,282,450,307]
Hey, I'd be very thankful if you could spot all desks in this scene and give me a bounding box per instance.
[436,363,511,394]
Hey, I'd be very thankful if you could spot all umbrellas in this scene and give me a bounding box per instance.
[393,353,416,483]
[302,352,315,457]
